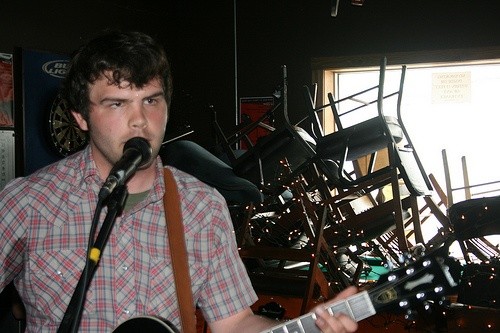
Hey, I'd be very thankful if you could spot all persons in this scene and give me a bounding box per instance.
[1,28,358,332]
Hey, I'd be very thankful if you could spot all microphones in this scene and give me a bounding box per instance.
[98,136,153,203]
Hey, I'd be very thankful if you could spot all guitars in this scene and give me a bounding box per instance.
[111,242,462,333]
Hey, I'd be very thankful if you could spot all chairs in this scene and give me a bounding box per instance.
[162,54,500,333]
